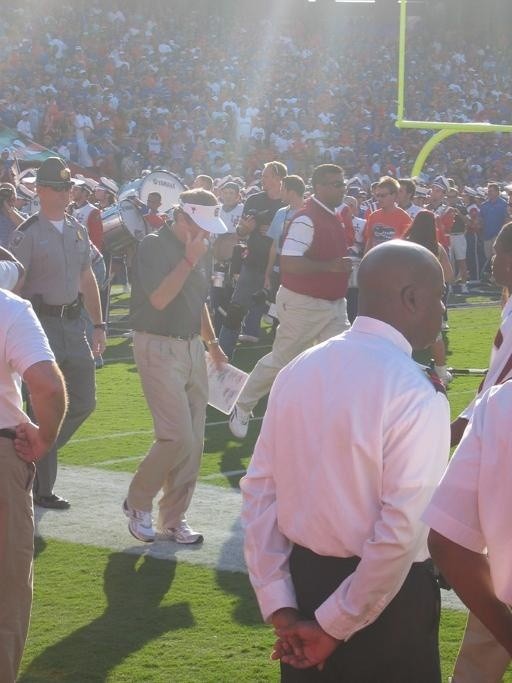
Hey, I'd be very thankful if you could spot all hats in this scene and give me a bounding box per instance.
[94,176,120,197]
[2,140,26,153]
[178,199,228,235]
[347,176,363,189]
[431,175,450,192]
[19,169,37,184]
[36,155,72,185]
[412,186,429,198]
[461,183,512,200]
[72,173,97,194]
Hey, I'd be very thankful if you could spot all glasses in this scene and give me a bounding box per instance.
[327,181,346,187]
[376,192,392,198]
[41,185,71,192]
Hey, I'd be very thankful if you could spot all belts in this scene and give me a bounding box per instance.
[450,233,463,236]
[137,328,201,342]
[1,428,18,441]
[38,298,81,317]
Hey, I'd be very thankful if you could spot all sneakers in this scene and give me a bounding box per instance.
[31,491,70,510]
[228,395,254,439]
[122,329,140,339]
[238,333,260,343]
[122,283,132,293]
[156,515,205,545]
[447,285,455,294]
[123,496,156,543]
[460,283,470,294]
